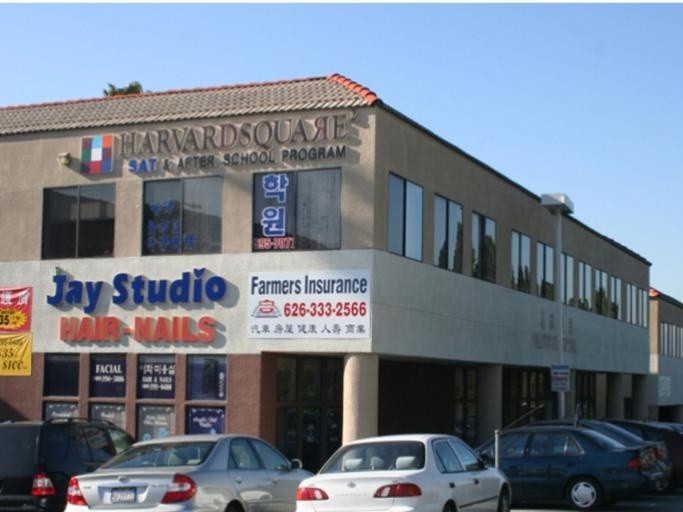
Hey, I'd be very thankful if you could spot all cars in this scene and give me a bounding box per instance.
[291,432,514,511]
[471,416,671,510]
[60,432,316,511]
[606,416,682,452]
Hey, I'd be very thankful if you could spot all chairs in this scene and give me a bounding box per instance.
[369,455,384,471]
[395,454,418,470]
[344,457,361,471]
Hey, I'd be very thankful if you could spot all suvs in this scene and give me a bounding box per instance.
[0,415,136,511]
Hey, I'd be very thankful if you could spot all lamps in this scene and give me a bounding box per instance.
[54,151,73,166]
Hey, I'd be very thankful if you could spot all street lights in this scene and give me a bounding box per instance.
[539,192,578,419]
